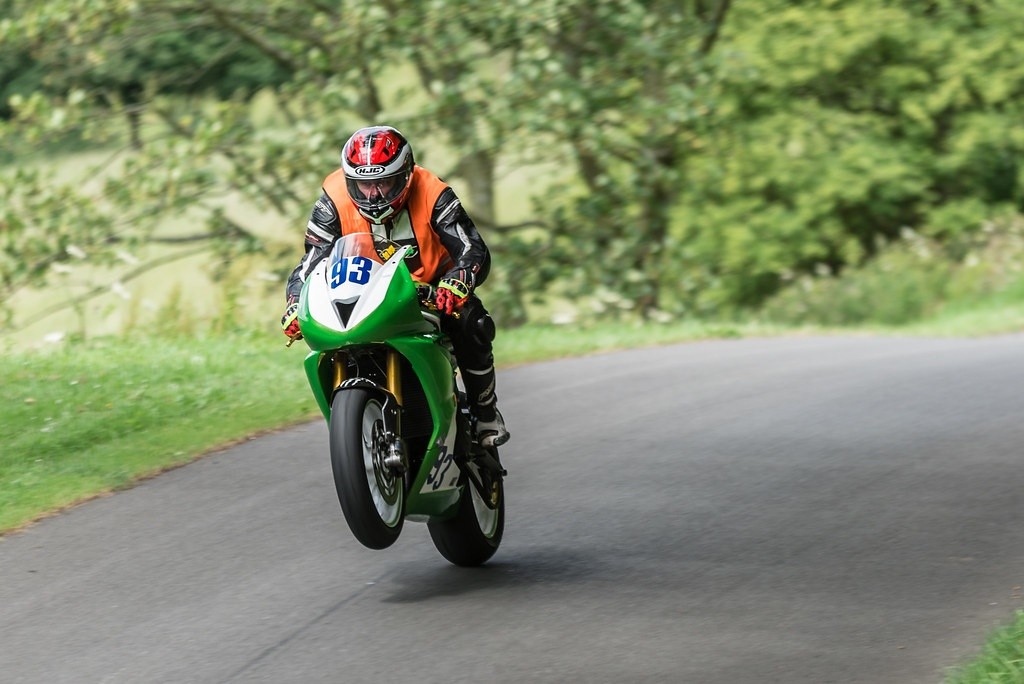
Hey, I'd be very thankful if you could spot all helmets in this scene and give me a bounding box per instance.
[340,126,415,223]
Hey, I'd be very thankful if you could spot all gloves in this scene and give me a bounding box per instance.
[436,269,476,315]
[281,295,304,340]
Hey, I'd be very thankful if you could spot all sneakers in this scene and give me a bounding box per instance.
[473,409,510,448]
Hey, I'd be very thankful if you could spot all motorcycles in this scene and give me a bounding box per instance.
[286,232,510,567]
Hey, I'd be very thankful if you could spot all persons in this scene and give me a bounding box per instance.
[280,124,511,449]
